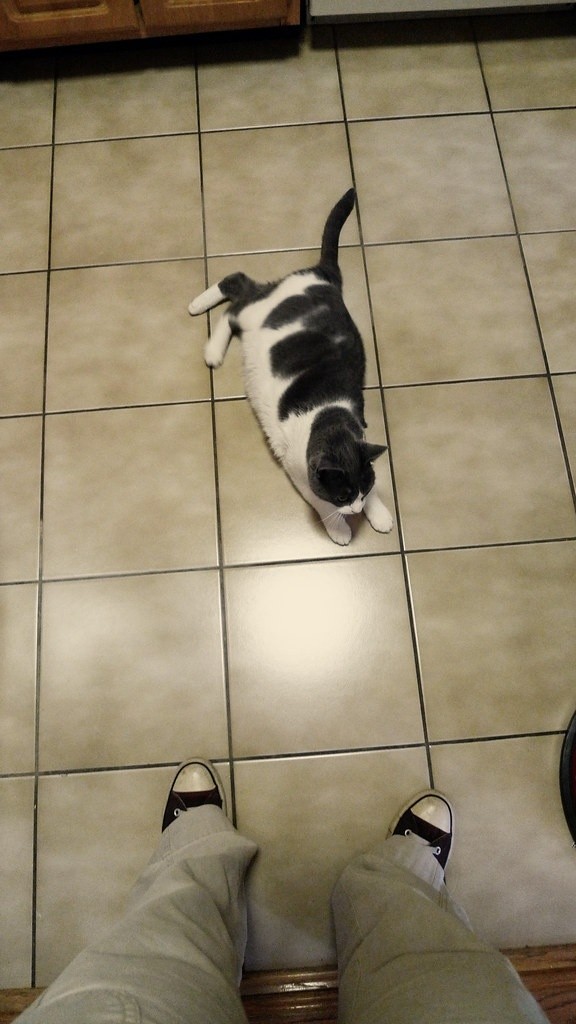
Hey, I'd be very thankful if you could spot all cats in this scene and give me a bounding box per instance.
[186,186,394,547]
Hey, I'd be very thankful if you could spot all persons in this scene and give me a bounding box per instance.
[11,757,551,1024]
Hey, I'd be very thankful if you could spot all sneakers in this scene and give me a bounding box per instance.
[387,789,455,870]
[161,758,227,838]
